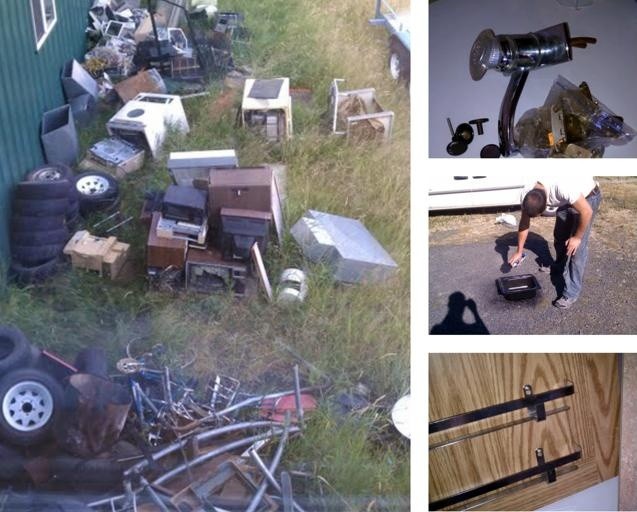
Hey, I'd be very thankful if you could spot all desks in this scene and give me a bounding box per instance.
[241,76,290,138]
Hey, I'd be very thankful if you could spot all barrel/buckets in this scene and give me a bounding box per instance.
[51,374,133,457]
[275,267,310,313]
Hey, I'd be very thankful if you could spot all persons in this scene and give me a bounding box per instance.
[507,174,602,309]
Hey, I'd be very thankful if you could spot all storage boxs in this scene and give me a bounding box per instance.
[106,91,190,160]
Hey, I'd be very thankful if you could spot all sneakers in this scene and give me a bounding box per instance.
[555,296,577,309]
[539,261,564,273]
